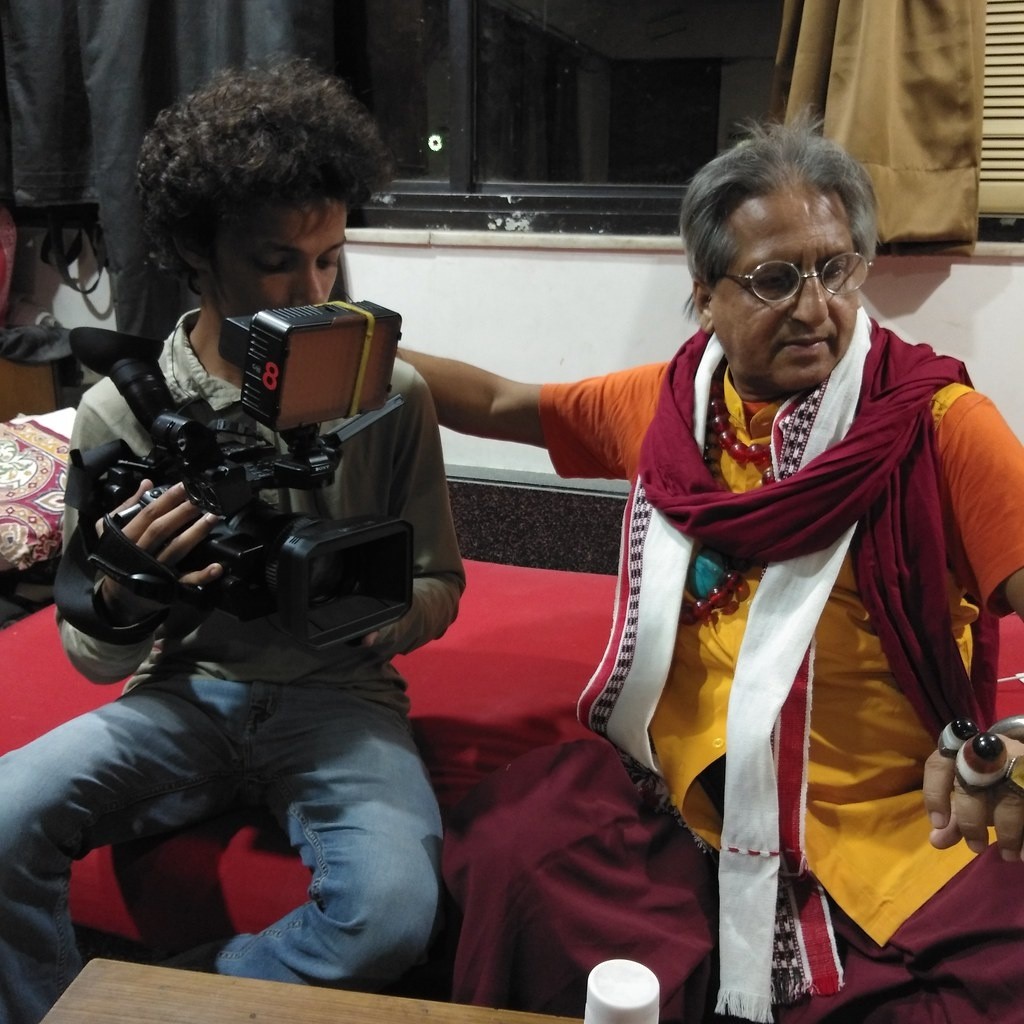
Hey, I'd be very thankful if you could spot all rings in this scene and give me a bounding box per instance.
[938,718,1023,795]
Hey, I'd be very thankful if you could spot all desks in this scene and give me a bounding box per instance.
[40,957,585,1024]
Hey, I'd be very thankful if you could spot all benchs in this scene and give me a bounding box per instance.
[1,461,1024,952]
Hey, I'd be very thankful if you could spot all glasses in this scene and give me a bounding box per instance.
[720,252,873,303]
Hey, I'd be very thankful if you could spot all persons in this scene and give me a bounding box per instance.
[393,121,1024,1024]
[0,56,467,1024]
